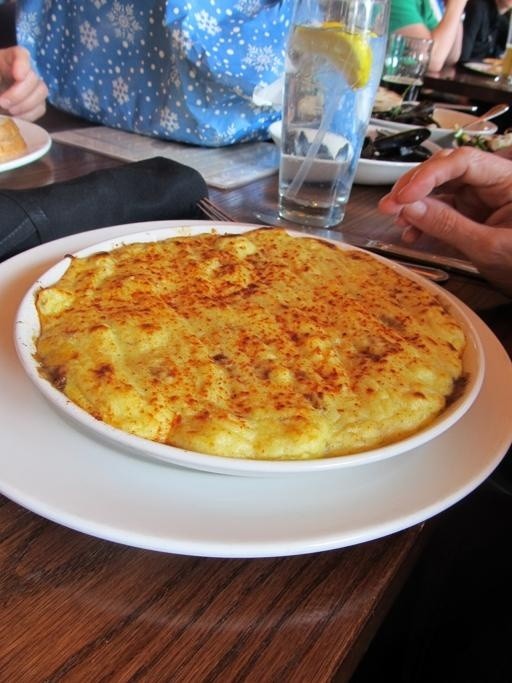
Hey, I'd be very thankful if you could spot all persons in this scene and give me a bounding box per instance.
[347,0,470,75]
[376,144,512,287]
[0,4,51,123]
[457,0,512,67]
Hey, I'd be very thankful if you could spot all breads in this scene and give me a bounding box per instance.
[0,117,27,162]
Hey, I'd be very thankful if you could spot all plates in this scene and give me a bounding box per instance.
[15,227,487,476]
[269,90,512,184]
[464,44,512,84]
[0,221,509,557]
[0,114,53,173]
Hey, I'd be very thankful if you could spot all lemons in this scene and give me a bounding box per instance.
[291,23,373,90]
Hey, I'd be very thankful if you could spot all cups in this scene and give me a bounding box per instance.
[277,0,390,226]
[385,32,433,87]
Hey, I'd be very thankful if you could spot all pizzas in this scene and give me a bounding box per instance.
[36,225,462,461]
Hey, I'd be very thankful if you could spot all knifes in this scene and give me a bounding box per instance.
[254,211,487,275]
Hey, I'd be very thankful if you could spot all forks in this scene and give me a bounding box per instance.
[196,198,448,282]
